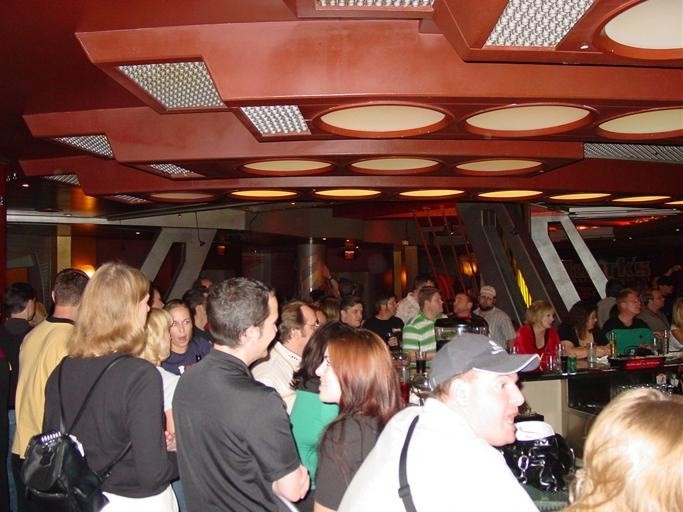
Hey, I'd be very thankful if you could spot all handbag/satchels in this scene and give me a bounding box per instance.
[503,419,575,494]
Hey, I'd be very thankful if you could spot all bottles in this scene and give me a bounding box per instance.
[662,329,669,355]
[650,338,657,352]
[510,346,516,355]
[608,330,616,359]
[614,384,675,397]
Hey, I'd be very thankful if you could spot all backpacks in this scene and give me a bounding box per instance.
[18,354,132,512]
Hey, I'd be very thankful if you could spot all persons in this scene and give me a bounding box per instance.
[1,260,683,512]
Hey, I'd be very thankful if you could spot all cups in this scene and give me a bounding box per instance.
[414,351,426,373]
[398,368,410,404]
[585,342,596,363]
[545,343,577,374]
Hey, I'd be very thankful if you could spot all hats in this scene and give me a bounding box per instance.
[425,333,541,393]
[480,286,496,296]
